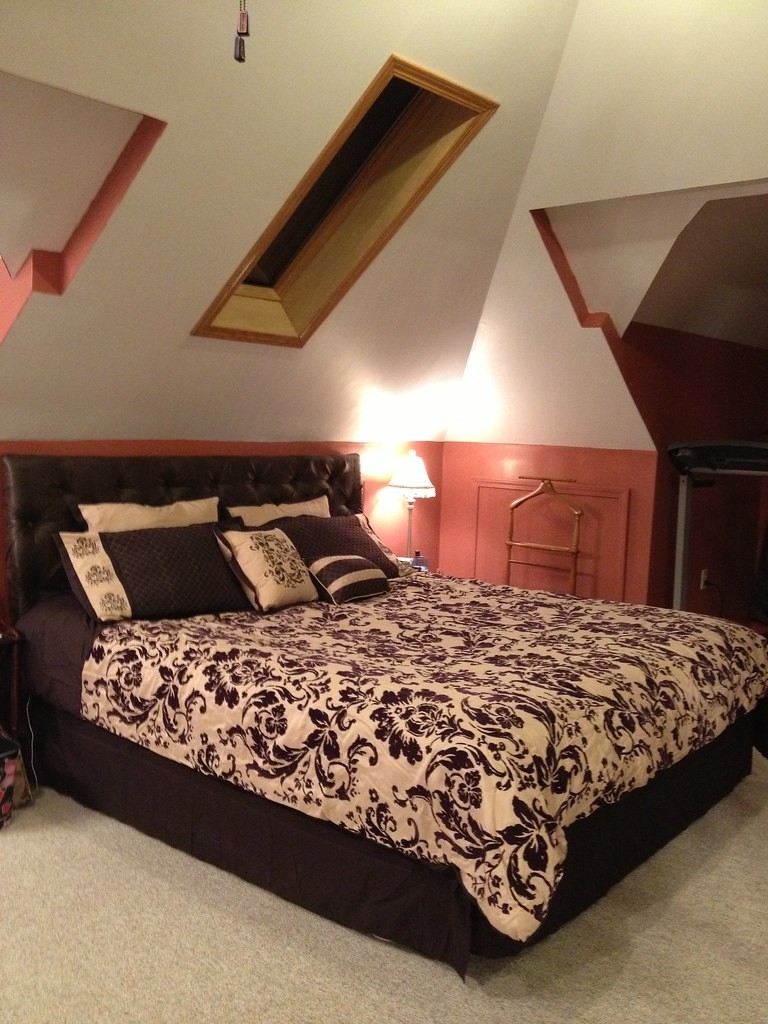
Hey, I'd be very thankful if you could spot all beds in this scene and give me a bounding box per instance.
[1,452,768,983]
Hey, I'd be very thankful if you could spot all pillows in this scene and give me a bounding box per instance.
[227,494,331,530]
[76,496,220,531]
[50,521,256,624]
[254,513,412,580]
[215,527,319,614]
[302,554,392,607]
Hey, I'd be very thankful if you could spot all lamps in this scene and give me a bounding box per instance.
[386,451,436,571]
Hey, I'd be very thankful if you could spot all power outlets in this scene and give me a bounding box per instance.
[699,569,708,590]
